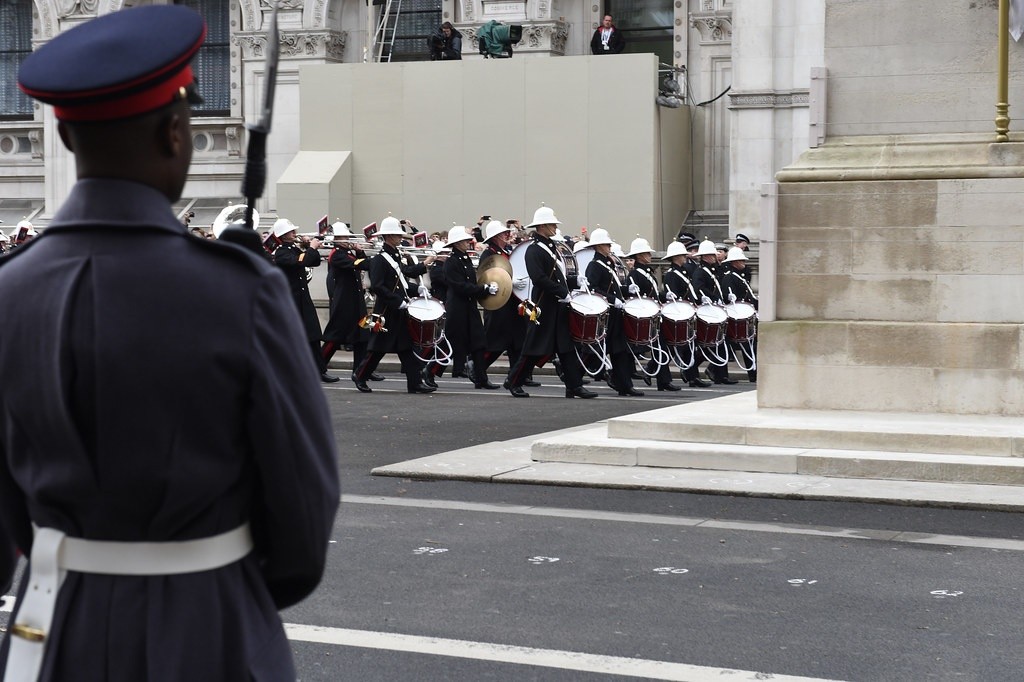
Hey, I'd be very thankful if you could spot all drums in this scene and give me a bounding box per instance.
[662,300,696,345]
[571,248,628,287]
[408,295,446,347]
[726,301,756,343]
[567,289,608,344]
[622,296,661,345]
[509,238,579,306]
[695,305,728,346]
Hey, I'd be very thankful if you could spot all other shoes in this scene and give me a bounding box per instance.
[322,374,340,382]
[476,381,500,389]
[680,367,688,383]
[619,387,644,397]
[642,367,650,385]
[452,370,468,378]
[503,379,530,397]
[566,386,598,398]
[351,372,372,393]
[689,378,711,387]
[422,371,439,388]
[658,383,681,390]
[408,384,434,394]
[524,377,542,386]
[714,376,737,385]
[369,373,384,381]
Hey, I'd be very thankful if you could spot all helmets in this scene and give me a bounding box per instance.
[721,247,749,264]
[692,240,720,256]
[443,225,473,248]
[482,221,514,244]
[585,228,613,249]
[332,221,351,234]
[626,238,655,257]
[523,207,561,228]
[372,217,406,235]
[661,241,690,260]
[273,218,299,238]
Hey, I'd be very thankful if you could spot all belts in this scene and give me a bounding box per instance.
[26,520,251,574]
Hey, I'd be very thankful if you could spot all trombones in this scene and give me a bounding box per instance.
[394,243,481,263]
[293,231,383,253]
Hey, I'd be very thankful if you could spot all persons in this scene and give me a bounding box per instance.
[502,203,600,397]
[0,216,758,398]
[438,22,462,60]
[0,5,342,682]
[591,14,626,54]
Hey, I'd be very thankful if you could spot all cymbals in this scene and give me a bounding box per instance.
[474,254,512,310]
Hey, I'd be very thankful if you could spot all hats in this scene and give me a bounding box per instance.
[736,234,750,244]
[20,6,209,120]
[7,221,38,237]
[678,233,695,241]
[685,240,700,250]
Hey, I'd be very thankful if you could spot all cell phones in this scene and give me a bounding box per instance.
[483,216,491,220]
[400,220,406,224]
